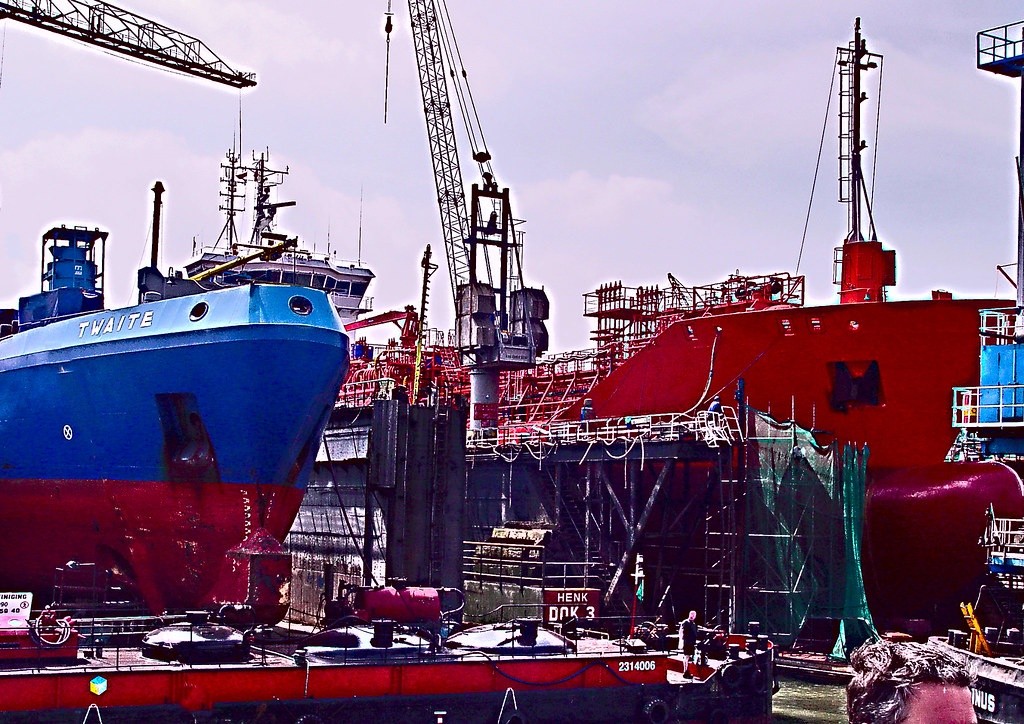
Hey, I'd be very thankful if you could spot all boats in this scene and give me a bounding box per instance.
[1,107,377,626]
[285,19,1024,653]
[0,585,780,724]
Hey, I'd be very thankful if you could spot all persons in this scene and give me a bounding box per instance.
[846,636,978,724]
[678,611,698,678]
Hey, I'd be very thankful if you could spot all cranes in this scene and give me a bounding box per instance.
[0,0,257,90]
[384,0,550,442]
